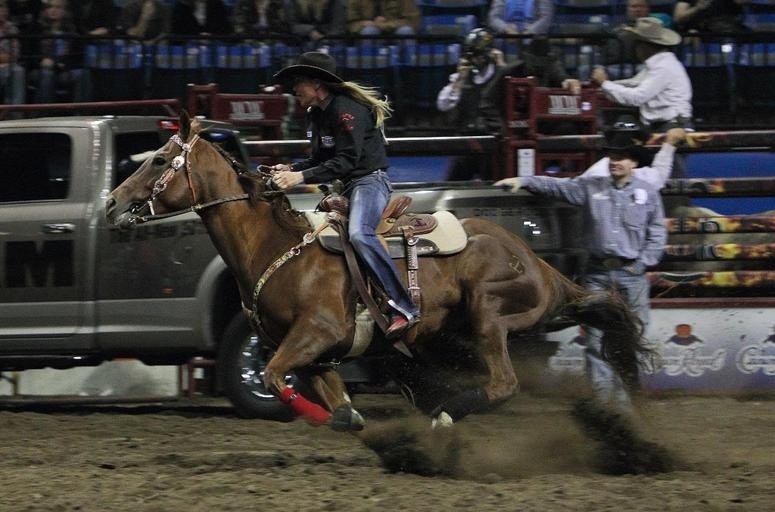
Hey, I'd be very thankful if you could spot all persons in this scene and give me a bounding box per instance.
[573,112,687,190]
[263,50,422,346]
[1,1,423,121]
[490,132,667,411]
[462,36,584,190]
[486,1,555,89]
[579,1,752,122]
[433,25,509,184]
[562,17,704,220]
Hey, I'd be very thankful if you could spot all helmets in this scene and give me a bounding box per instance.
[463,28,494,55]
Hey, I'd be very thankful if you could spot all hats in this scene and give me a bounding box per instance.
[602,137,649,168]
[621,17,682,45]
[272,51,344,89]
[605,113,651,141]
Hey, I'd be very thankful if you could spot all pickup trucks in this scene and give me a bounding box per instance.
[0,108,564,421]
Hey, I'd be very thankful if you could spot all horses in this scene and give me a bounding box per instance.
[106,103,672,476]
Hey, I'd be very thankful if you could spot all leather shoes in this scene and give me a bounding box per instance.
[385,312,421,340]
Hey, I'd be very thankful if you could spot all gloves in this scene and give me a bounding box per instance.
[272,163,304,188]
[493,177,522,194]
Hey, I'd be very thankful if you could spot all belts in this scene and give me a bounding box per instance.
[590,255,632,269]
